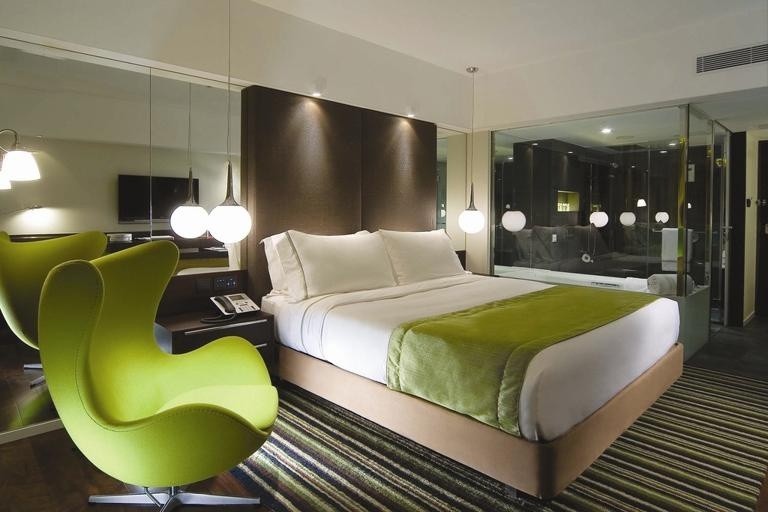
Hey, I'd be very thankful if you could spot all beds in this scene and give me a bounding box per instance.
[261,273,684,501]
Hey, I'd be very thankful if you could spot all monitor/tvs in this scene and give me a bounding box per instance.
[117,174,199,223]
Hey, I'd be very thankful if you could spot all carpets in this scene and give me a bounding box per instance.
[230,364,768,512]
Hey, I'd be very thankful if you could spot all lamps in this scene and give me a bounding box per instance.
[0,129,40,182]
[655,211,670,224]
[620,212,636,227]
[170,83,208,239]
[501,189,527,233]
[206,0,251,245]
[589,211,609,228]
[0,171,11,190]
[636,198,647,208]
[457,67,485,236]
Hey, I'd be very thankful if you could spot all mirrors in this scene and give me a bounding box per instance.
[0,36,242,448]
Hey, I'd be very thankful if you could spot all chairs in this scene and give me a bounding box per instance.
[38,239,278,511]
[0,230,109,389]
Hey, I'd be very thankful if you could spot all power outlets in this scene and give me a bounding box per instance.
[214,275,239,294]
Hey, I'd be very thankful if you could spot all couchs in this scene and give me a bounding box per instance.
[513,252,662,280]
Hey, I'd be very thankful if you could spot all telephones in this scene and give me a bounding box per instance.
[201,292,260,323]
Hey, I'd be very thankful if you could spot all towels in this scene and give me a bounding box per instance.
[661,227,693,272]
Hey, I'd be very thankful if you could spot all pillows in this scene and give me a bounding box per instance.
[258,236,288,296]
[272,229,397,302]
[533,225,574,263]
[566,223,609,254]
[512,229,538,263]
[377,228,466,285]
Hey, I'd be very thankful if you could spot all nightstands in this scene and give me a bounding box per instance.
[154,311,279,387]
